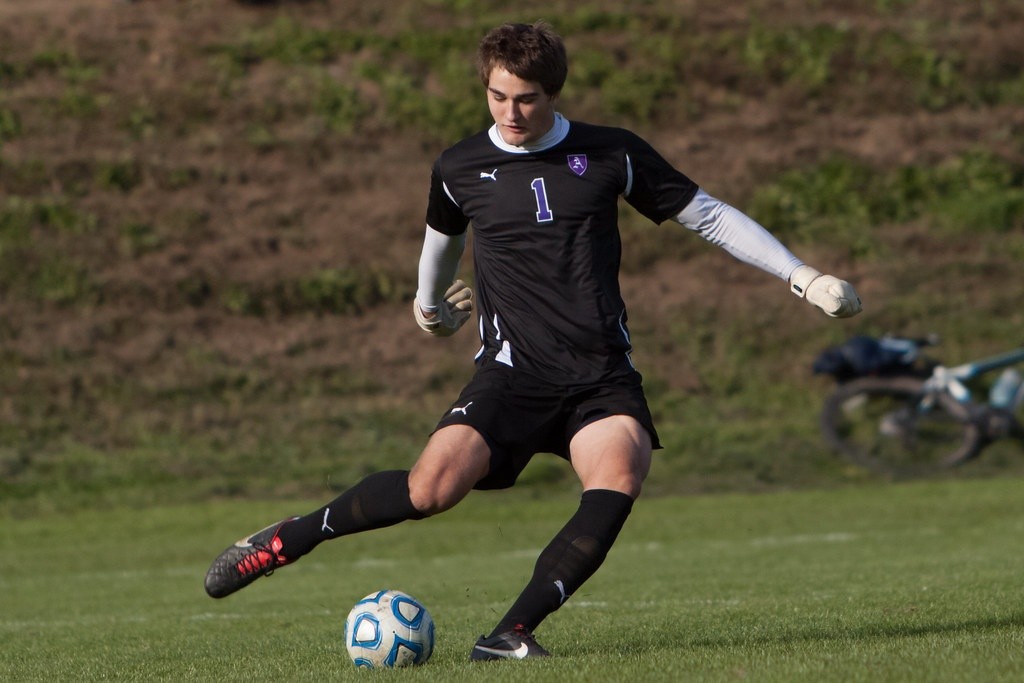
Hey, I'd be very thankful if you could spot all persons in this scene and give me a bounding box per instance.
[205,21,863,660]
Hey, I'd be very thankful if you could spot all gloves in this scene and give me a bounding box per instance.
[413,280,475,338]
[787,265,864,316]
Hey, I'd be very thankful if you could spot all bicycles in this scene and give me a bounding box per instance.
[814,334,1024,478]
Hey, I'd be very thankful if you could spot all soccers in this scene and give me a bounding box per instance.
[344,589,435,670]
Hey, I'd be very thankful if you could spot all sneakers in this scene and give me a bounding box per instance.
[203,515,306,600]
[467,625,551,662]
